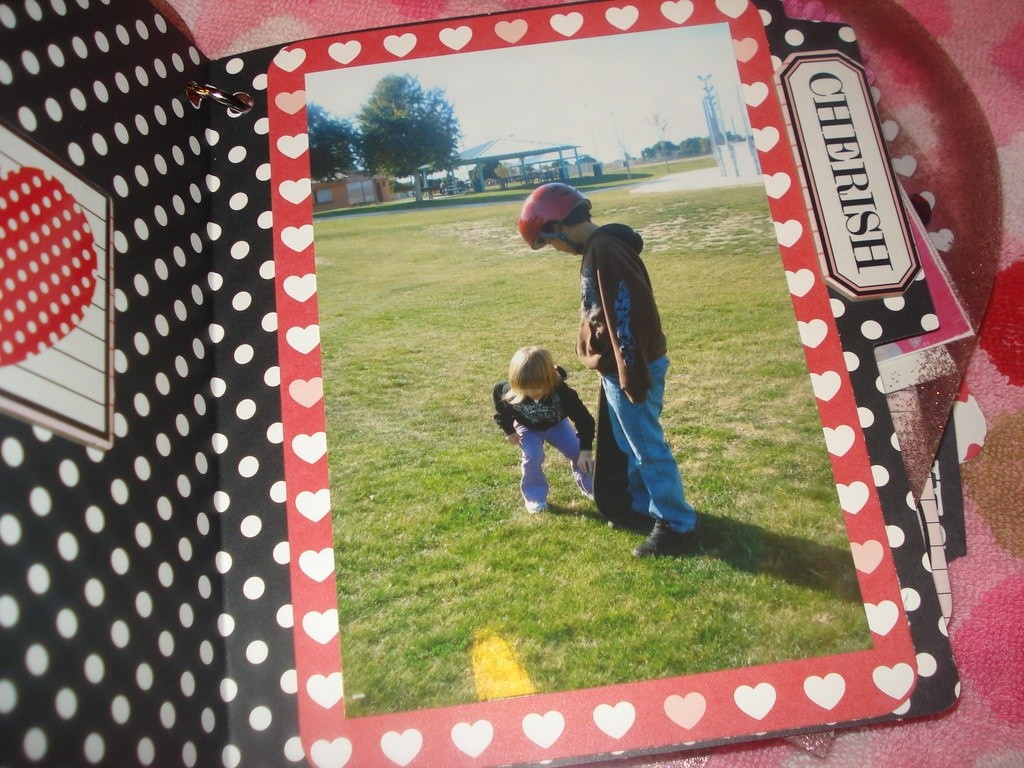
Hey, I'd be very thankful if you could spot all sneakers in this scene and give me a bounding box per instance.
[632,520,696,558]
[608,511,656,529]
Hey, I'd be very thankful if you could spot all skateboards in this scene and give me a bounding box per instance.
[591,378,645,524]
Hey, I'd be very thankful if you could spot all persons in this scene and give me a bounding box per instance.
[492,348,595,515]
[519,183,696,555]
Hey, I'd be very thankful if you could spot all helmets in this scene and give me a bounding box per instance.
[519,183,592,251]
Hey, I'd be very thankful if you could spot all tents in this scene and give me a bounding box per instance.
[418,138,583,199]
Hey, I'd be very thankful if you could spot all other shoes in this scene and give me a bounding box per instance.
[524,498,548,513]
